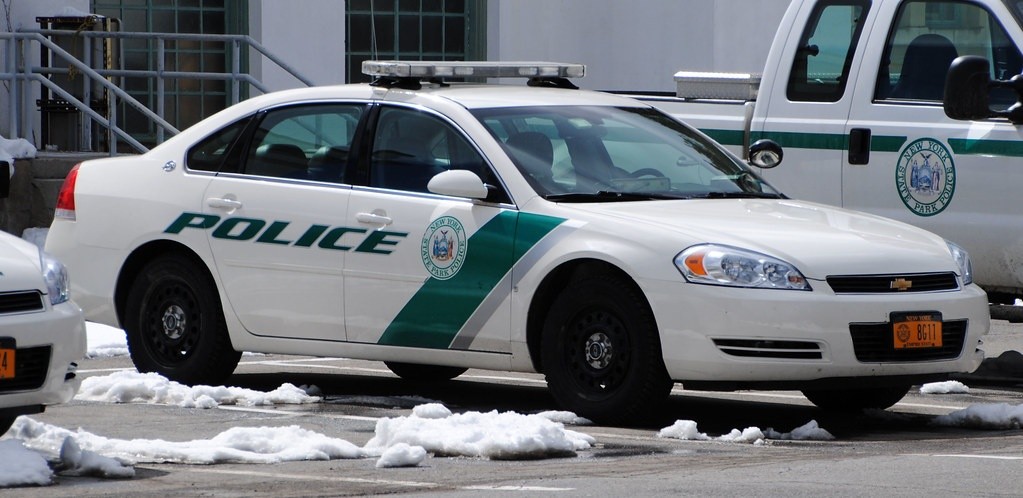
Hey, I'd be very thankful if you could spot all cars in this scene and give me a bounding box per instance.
[43,60,990,426]
[0,227,87,439]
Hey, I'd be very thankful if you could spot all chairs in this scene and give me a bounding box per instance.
[890,34,959,99]
[307,146,351,182]
[256,144,308,180]
[384,137,447,193]
[505,132,566,195]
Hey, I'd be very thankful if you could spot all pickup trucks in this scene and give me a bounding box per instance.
[485,0,1023,301]
